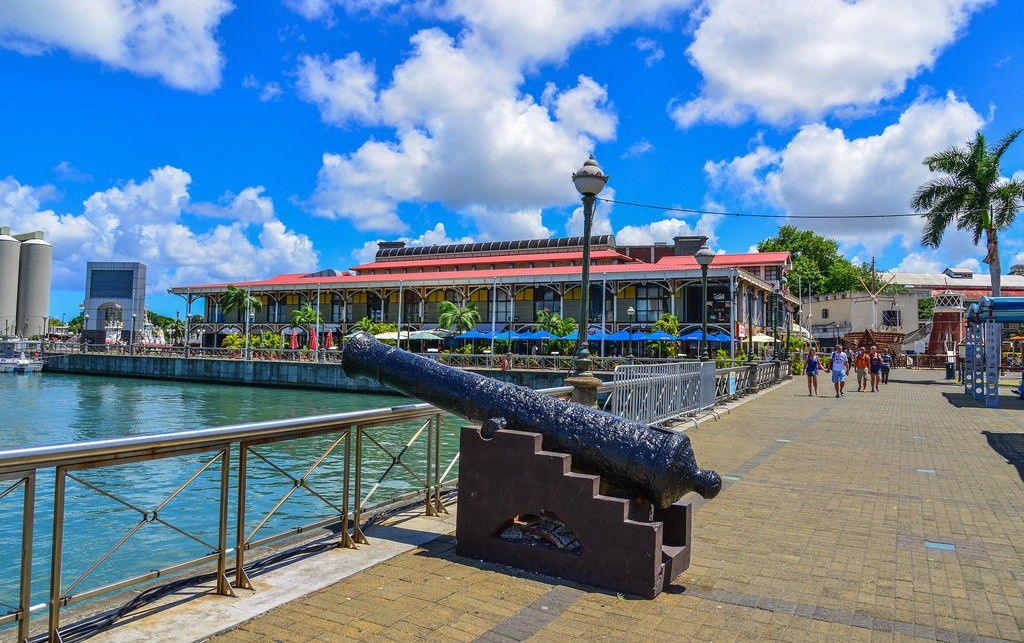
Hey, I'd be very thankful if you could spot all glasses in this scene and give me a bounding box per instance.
[811,350,816,351]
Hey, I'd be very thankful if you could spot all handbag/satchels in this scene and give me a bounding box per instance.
[830,352,836,371]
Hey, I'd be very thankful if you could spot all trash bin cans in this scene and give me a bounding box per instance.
[944,362,955,379]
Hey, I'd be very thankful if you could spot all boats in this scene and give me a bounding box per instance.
[840,256,906,356]
[0,320,45,373]
[105,308,173,351]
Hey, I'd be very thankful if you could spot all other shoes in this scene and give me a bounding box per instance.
[1011,390,1019,394]
[835,395,840,398]
[840,392,845,396]
[1016,397,1024,399]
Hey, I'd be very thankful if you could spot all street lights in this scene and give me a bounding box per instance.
[167,328,176,350]
[197,328,206,356]
[85,314,90,342]
[627,306,635,357]
[571,155,610,379]
[186,313,193,341]
[695,243,715,362]
[132,313,137,343]
[43,316,48,341]
[250,312,255,345]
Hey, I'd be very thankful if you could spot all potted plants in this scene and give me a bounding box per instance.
[222,332,288,360]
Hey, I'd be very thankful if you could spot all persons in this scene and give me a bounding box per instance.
[854,347,870,392]
[880,349,892,385]
[844,346,855,371]
[868,345,883,392]
[802,347,828,396]
[891,350,897,370]
[531,342,540,355]
[140,336,146,353]
[118,338,127,355]
[826,344,850,398]
[1006,356,1024,400]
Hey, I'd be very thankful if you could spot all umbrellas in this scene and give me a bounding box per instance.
[373,328,781,359]
[309,327,316,351]
[325,328,334,350]
[290,327,299,349]
